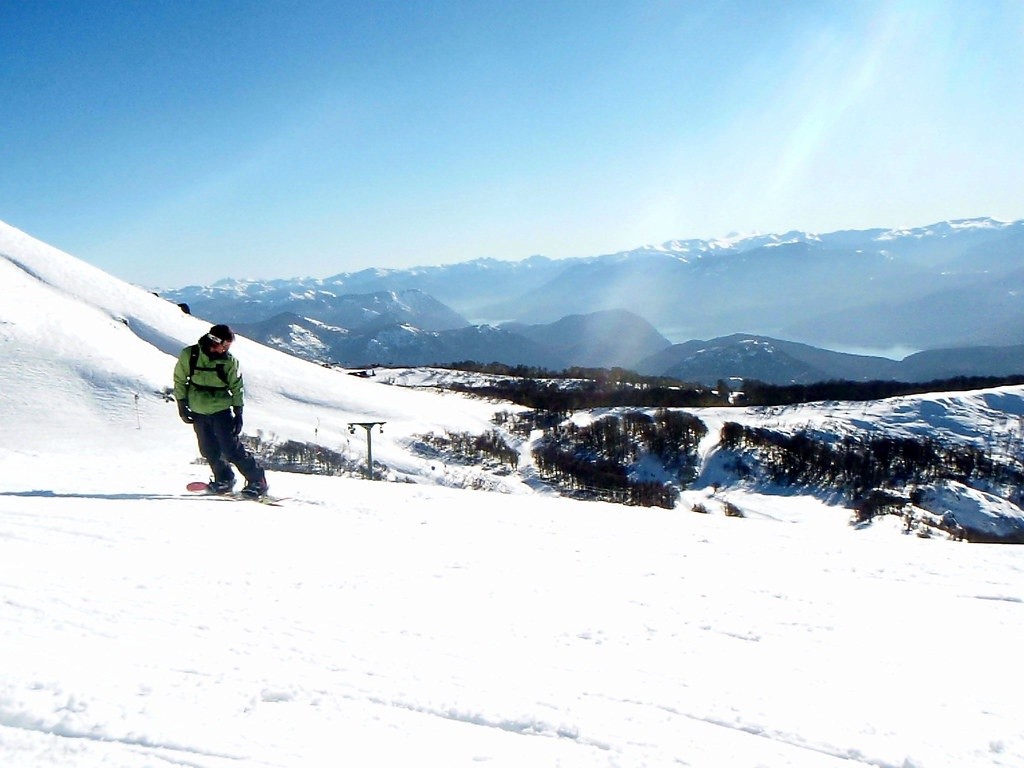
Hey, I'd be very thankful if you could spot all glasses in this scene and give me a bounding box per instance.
[207,333,232,347]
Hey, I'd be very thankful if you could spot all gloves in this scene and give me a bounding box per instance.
[176,399,195,424]
[230,407,243,435]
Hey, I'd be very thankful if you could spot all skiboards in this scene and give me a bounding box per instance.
[187,481,318,508]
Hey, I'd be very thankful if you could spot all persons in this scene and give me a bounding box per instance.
[174,325,268,497]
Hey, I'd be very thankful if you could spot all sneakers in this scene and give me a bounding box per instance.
[210,475,237,492]
[241,467,269,497]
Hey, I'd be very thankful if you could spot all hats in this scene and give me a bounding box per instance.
[199,325,235,348]
